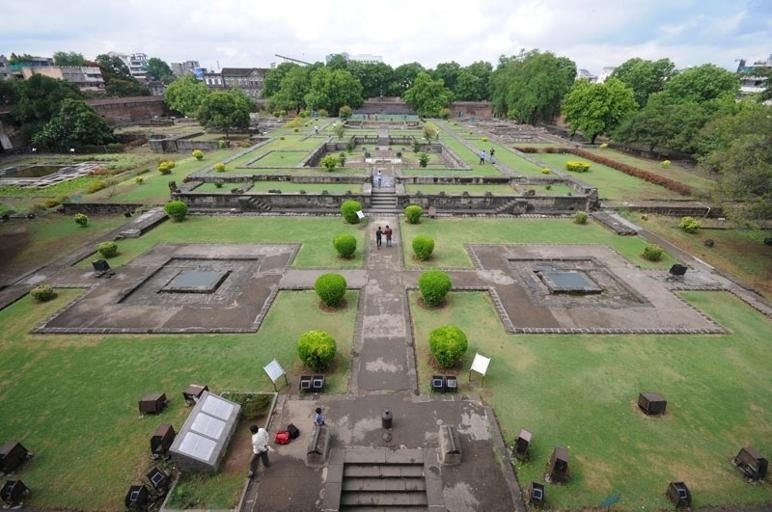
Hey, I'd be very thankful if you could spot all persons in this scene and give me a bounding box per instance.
[478,150,486,165]
[375,169,383,190]
[488,147,494,156]
[488,152,496,165]
[245,424,272,479]
[314,407,327,427]
[385,225,392,247]
[376,226,385,249]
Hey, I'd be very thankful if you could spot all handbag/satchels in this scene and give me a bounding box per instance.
[287,424,299,438]
[275,431,291,444]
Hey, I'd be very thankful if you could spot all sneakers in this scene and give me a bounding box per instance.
[245,473,254,478]
